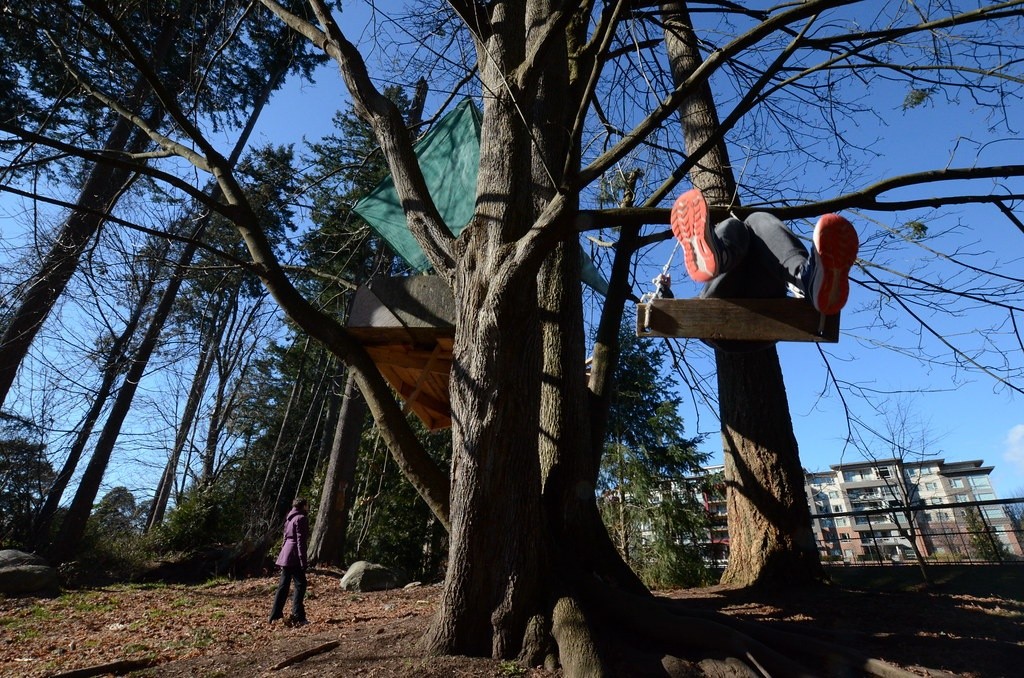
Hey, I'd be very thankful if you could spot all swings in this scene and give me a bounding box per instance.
[635,202,841,344]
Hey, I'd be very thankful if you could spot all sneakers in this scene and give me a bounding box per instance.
[670,188,722,284]
[800,214,860,316]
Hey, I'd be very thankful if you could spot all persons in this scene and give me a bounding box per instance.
[657,190,860,355]
[268,497,310,624]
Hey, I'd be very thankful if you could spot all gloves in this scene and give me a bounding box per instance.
[300,565,307,570]
